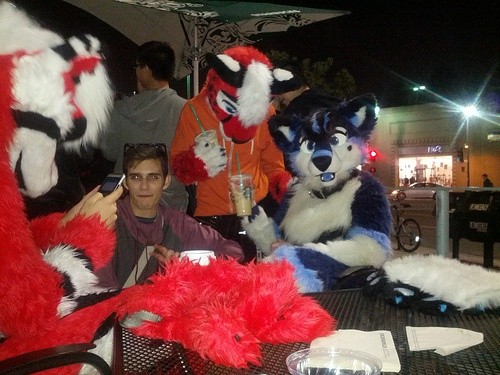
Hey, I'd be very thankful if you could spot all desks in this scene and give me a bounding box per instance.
[109,282,500,375]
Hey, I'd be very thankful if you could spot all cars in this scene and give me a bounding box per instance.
[389,181,452,201]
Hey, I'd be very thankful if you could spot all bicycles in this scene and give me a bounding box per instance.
[391,203,422,253]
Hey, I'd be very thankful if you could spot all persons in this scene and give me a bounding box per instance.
[481,174,494,187]
[171,45,394,293]
[0,0,244,375]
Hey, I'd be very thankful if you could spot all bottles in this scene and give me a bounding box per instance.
[400,161,451,186]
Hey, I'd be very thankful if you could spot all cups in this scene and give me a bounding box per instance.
[285,347,383,375]
[229,174,253,216]
[195,129,226,168]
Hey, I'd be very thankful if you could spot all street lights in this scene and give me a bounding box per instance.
[461,105,475,188]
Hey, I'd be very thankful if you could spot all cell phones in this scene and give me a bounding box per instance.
[97,172,125,197]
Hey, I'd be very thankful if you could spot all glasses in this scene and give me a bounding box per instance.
[123,143,169,174]
[132,63,141,71]
